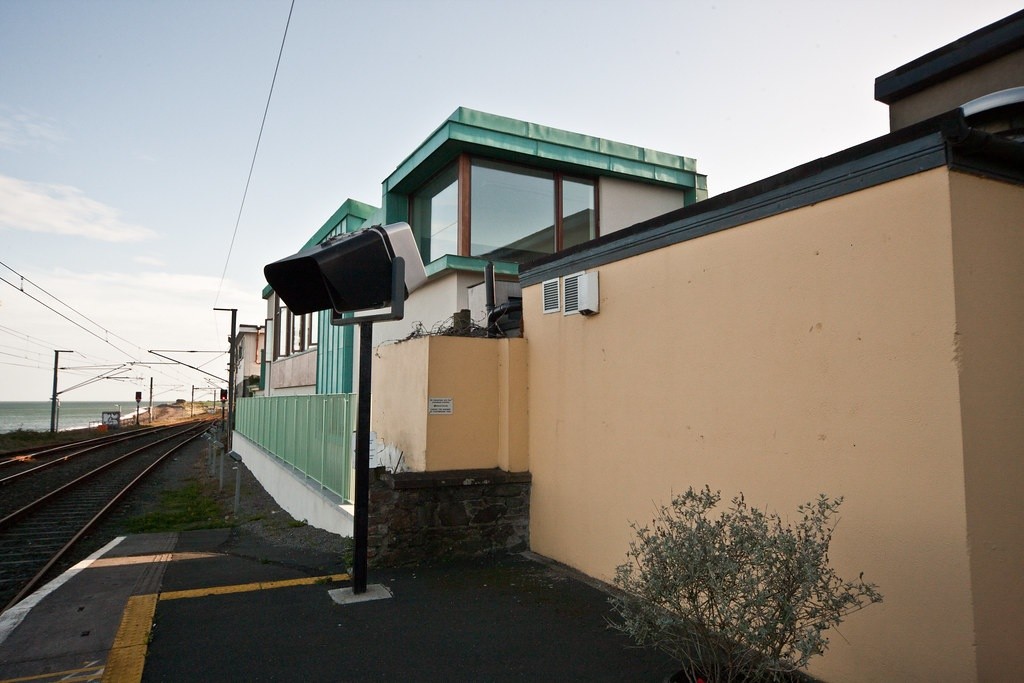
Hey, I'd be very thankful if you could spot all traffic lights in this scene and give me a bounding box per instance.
[221,388,227,402]
